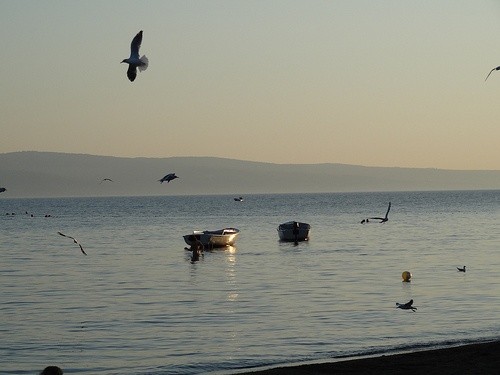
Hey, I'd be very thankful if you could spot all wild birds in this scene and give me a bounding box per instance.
[58,232,87,255]
[395,299,417,313]
[119,30,149,82]
[368,201,392,223]
[484,66,500,80]
[158,173,179,184]
[456,266,466,272]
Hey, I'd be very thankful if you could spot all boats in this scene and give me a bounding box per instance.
[183,227,240,249]
[276,222,312,241]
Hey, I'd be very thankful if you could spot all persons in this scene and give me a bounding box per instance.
[184,235,204,257]
[40,366,63,375]
